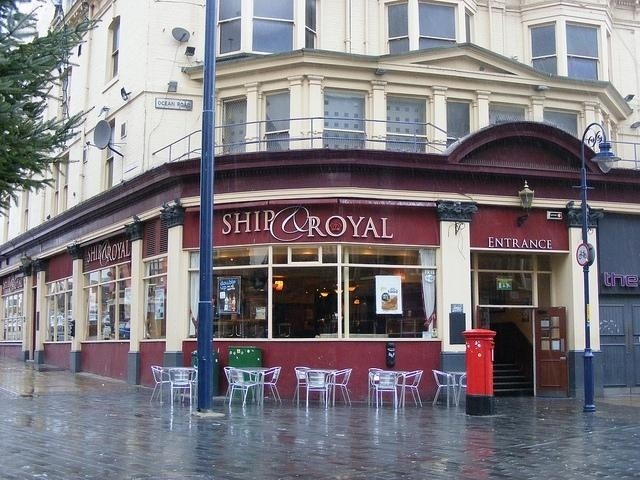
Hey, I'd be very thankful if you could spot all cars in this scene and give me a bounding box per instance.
[5,311,130,339]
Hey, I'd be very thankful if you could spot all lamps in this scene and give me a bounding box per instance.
[516,179,537,228]
[120,87,133,102]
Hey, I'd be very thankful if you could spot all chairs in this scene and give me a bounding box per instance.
[224,367,282,407]
[151,366,198,416]
[432,370,465,408]
[292,367,352,410]
[368,368,424,408]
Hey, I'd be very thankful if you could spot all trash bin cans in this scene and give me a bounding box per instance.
[191,350,220,396]
[226,346,263,398]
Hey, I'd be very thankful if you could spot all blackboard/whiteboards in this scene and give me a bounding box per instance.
[217,276,242,314]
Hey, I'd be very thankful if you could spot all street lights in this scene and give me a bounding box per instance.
[574,124,621,411]
